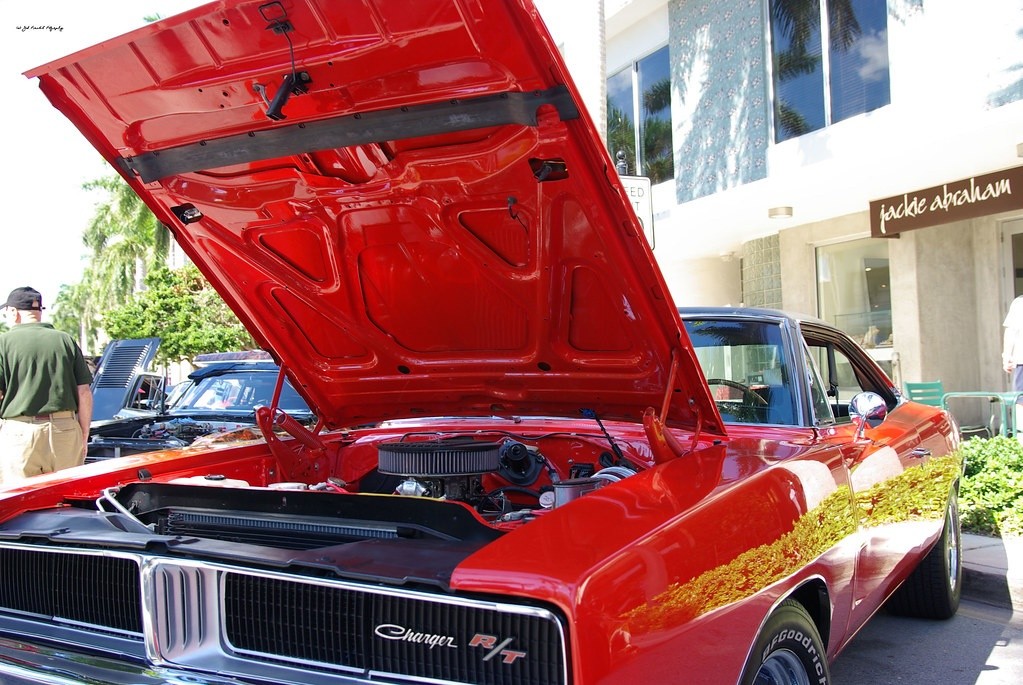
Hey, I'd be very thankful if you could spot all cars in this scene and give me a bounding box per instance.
[0,0,962,685]
[81,335,319,466]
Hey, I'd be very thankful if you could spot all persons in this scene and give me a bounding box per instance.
[1002,295,1023,406]
[0,286,94,487]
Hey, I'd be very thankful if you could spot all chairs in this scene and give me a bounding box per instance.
[740,386,822,426]
[903,380,992,442]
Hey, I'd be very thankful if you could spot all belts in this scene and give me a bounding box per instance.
[8,410,73,422]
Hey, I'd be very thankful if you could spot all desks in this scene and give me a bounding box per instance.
[940,389,1023,440]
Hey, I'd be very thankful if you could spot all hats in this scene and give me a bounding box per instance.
[0,287,46,311]
